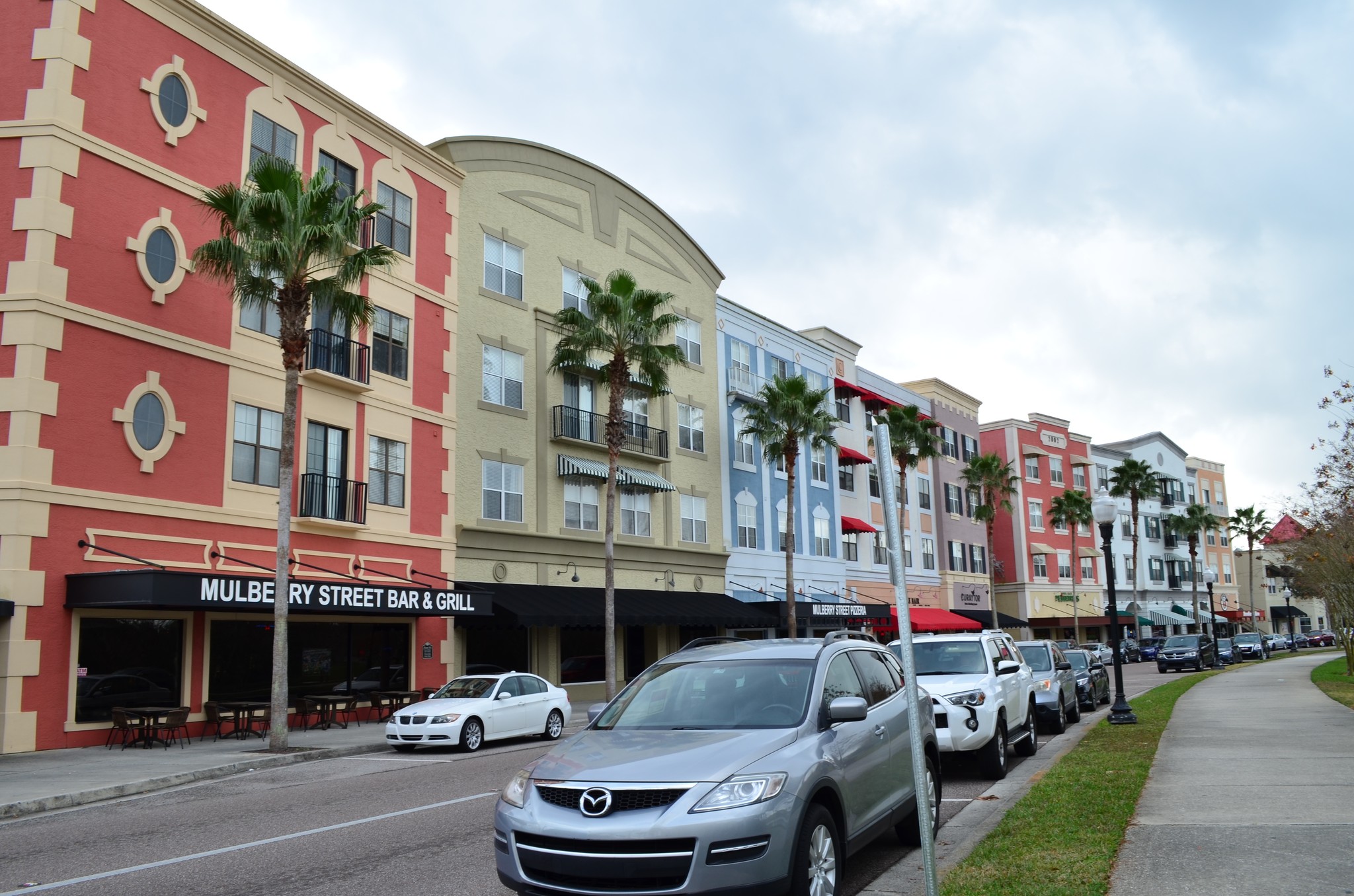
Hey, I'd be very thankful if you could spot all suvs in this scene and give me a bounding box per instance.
[1233,632,1273,659]
[1009,639,1082,734]
[493,631,942,896]
[1306,629,1336,647]
[884,628,1045,779]
[1157,633,1215,673]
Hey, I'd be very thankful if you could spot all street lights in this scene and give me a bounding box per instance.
[1283,587,1299,653]
[1091,486,1140,722]
[1201,565,1231,670]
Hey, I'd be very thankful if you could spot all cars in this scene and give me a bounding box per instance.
[384,671,571,753]
[1137,637,1168,661]
[1105,638,1142,665]
[1264,633,1288,650]
[1211,639,1244,665]
[1079,644,1114,666]
[1061,650,1113,710]
[1281,633,1310,649]
[1331,627,1354,644]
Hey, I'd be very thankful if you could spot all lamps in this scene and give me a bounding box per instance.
[923,587,938,598]
[1183,598,1194,606]
[794,584,803,594]
[1078,591,1086,597]
[557,561,580,582]
[1053,591,1063,597]
[977,584,989,594]
[1164,596,1174,605]
[1223,595,1228,602]
[907,586,923,599]
[823,587,836,595]
[962,583,975,595]
[748,582,763,593]
[1234,600,1237,604]
[1148,597,1158,605]
[1198,598,1207,605]
[655,570,675,587]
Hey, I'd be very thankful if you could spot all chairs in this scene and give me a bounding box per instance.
[366,690,422,724]
[200,701,271,743]
[105,706,192,752]
[424,686,447,699]
[291,695,360,732]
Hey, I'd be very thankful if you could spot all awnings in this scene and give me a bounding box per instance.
[63,540,494,618]
[1149,605,1227,626]
[1164,553,1187,562]
[841,516,876,532]
[557,453,676,493]
[454,581,780,627]
[1078,546,1103,557]
[558,349,673,397]
[1210,601,1266,621]
[1157,471,1180,482]
[834,377,942,427]
[873,607,982,633]
[838,446,872,464]
[1280,565,1303,577]
[1028,604,1135,627]
[1161,512,1177,520]
[1270,606,1308,618]
[1105,610,1155,626]
[949,609,1031,629]
[729,582,892,627]
[1266,565,1286,577]
[1030,543,1058,554]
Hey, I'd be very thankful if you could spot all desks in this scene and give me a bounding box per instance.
[305,695,354,730]
[217,702,271,740]
[120,707,179,749]
[377,691,420,721]
